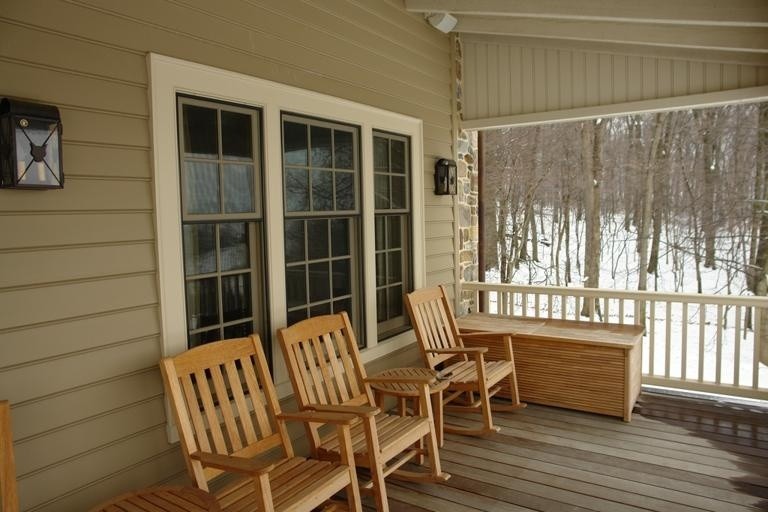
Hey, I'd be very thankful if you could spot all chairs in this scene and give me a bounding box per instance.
[160,333,363,512]
[404,284,528,436]
[277,310,451,512]
[0,399,21,512]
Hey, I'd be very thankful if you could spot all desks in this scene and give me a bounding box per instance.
[370,368,450,465]
[90,484,221,512]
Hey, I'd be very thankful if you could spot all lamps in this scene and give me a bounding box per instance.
[433,159,458,196]
[0,98,64,191]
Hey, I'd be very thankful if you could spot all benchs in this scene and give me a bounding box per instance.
[439,312,648,423]
